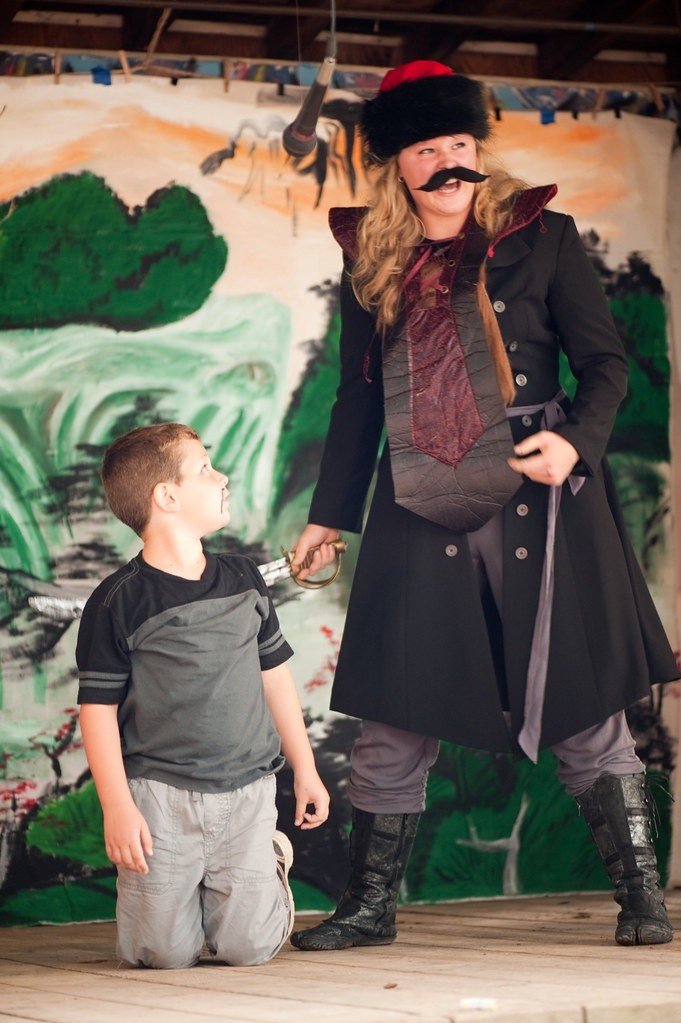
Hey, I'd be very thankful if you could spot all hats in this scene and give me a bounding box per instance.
[361,60,492,163]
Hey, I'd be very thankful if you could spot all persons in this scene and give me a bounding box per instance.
[74,422,331,968]
[290,60,680,950]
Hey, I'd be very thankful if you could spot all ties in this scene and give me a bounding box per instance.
[399,214,483,467]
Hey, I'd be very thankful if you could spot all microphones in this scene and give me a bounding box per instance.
[282,57,335,158]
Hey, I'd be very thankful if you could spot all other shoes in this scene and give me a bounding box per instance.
[271,830,296,941]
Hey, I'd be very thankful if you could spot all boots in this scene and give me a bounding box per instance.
[290,805,421,949]
[575,772,676,945]
